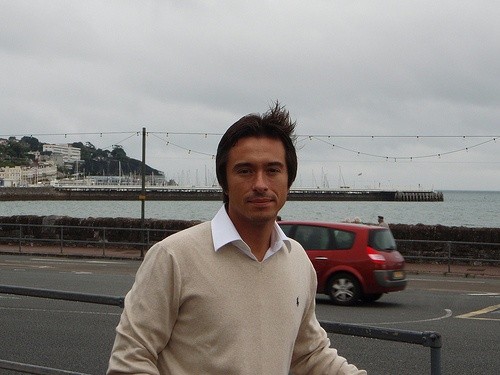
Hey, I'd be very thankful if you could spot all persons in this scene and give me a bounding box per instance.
[377,216,389,228]
[276,216,282,221]
[104,113,368,375]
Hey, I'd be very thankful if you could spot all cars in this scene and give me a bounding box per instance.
[276,220,406,305]
